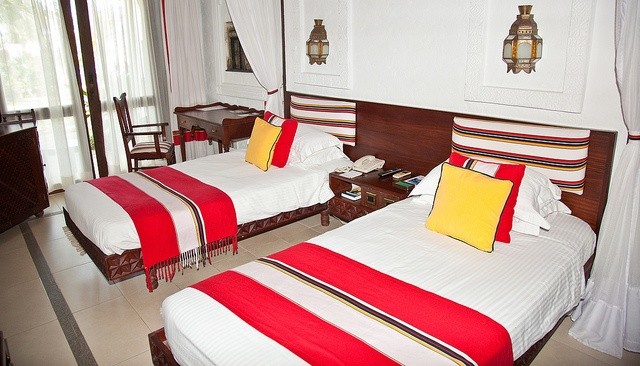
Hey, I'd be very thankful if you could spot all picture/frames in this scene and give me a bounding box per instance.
[464,1,594,113]
[286,1,352,90]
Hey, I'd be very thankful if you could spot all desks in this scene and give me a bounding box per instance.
[173,102,265,162]
[0,110,49,235]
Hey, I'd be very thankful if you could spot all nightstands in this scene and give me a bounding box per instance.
[329,169,412,222]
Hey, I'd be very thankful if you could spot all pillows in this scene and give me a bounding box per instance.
[426,162,513,252]
[247,123,343,167]
[297,145,349,167]
[408,157,562,231]
[449,152,526,244]
[245,117,282,172]
[262,111,298,168]
[512,200,572,237]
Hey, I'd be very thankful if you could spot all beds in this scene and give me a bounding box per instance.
[149,197,598,366]
[63,149,329,285]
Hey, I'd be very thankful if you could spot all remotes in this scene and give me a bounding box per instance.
[393,170,411,179]
[378,166,402,177]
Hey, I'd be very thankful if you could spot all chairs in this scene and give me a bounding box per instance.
[113,93,176,173]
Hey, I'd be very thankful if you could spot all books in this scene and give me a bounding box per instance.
[341,190,362,200]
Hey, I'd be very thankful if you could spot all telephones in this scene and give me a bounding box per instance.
[352,155,385,173]
